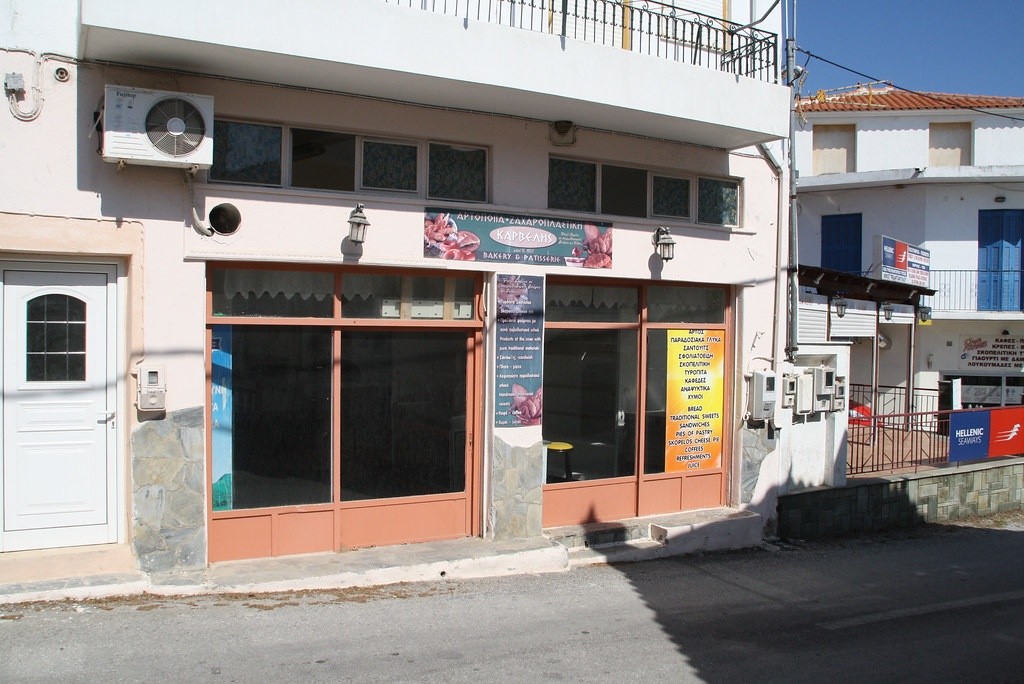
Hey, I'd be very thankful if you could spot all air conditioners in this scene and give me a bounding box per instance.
[103,84,214,170]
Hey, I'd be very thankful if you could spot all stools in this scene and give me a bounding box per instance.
[546,442,575,482]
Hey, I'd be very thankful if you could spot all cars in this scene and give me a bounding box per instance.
[849,401,883,427]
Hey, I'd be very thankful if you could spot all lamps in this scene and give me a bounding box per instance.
[829,295,847,318]
[651,227,677,263]
[346,202,370,246]
[878,296,895,321]
[915,303,930,322]
